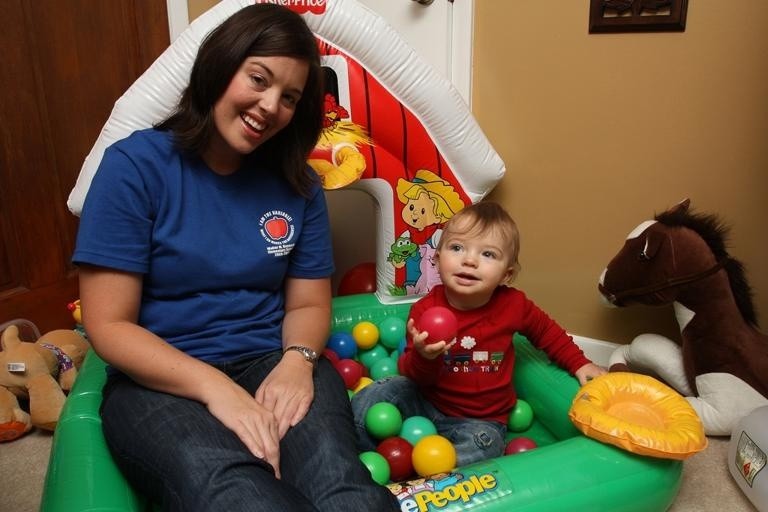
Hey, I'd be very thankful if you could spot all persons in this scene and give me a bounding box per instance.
[72,1,399,510]
[362,201,610,476]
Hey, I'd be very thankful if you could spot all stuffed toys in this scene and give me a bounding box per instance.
[594,200,767,402]
[0,325,91,443]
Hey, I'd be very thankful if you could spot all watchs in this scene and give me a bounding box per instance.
[280,344,320,367]
